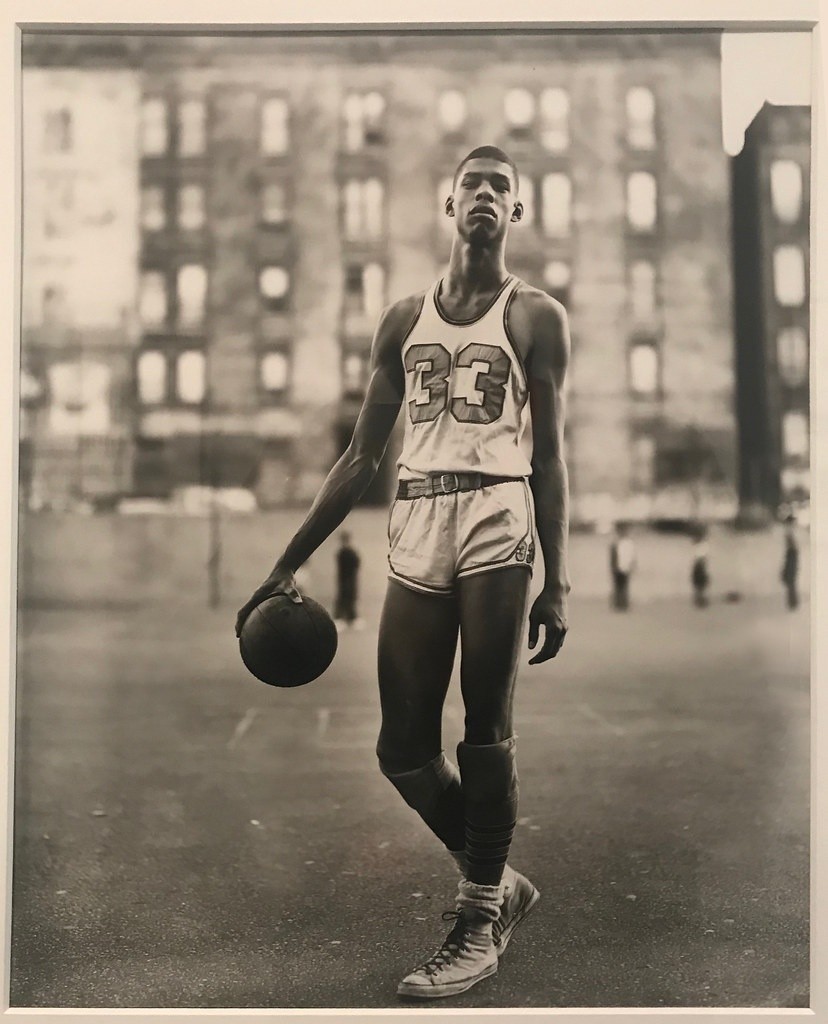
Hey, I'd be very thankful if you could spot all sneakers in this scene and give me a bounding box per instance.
[491,871,541,956]
[396,910,498,998]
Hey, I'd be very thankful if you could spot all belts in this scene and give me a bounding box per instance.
[395,470,526,500]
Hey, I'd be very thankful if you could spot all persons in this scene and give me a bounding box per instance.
[610,520,634,611]
[778,513,799,612]
[235,145,572,998]
[332,529,361,630]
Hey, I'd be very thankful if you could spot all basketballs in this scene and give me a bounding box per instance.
[237,592,340,690]
